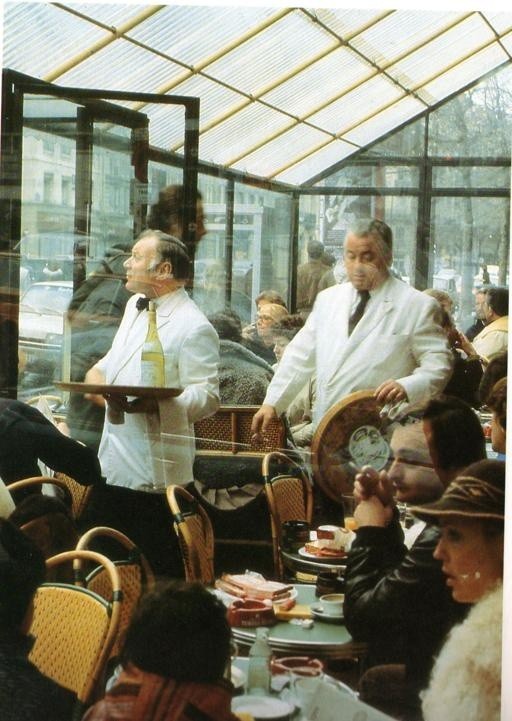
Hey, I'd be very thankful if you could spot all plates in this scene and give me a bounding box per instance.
[297,544,349,560]
[309,603,346,622]
[228,694,296,720]
[212,577,299,609]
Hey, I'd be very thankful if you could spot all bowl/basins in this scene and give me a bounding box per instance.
[319,592,344,613]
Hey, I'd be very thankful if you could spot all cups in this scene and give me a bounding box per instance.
[288,666,325,711]
[339,490,364,532]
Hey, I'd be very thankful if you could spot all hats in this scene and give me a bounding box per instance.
[410,458,504,525]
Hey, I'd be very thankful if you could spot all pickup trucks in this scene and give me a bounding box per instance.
[2,231,127,286]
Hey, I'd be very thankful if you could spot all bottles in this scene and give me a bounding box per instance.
[140,301,166,388]
[247,627,273,694]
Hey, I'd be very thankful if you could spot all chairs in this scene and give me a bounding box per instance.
[186,406,309,566]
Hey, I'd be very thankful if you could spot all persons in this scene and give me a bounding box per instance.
[252,217,454,446]
[0,227,510,720]
[64,185,206,449]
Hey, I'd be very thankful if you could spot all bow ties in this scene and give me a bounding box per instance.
[136,297,150,311]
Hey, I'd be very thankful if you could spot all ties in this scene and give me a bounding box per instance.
[349,290,370,337]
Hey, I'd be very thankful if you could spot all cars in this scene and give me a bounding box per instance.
[474,264,508,290]
[67,270,262,383]
[19,278,112,381]
[430,274,457,299]
[436,269,461,287]
